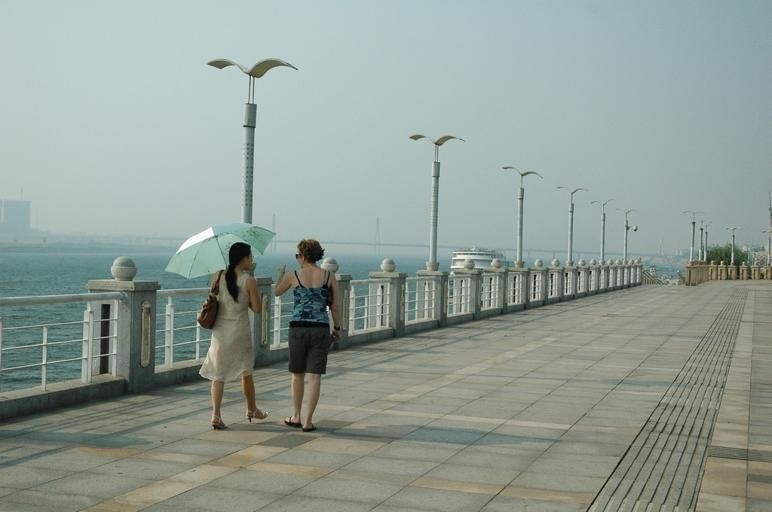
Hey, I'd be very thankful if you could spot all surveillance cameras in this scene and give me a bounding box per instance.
[633,225,638,231]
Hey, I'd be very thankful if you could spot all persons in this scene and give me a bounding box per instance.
[272,237,342,433]
[198,242,271,429]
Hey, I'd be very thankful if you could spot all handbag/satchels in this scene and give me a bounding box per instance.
[197,269,224,330]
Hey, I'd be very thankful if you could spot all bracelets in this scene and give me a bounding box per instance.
[333,325,341,331]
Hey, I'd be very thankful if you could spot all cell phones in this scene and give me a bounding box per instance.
[329,339,334,350]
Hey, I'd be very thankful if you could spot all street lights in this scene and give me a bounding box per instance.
[207,57,299,224]
[725,225,744,265]
[616,208,636,260]
[590,198,615,260]
[555,186,586,266]
[682,208,713,262]
[410,134,465,271]
[502,166,543,267]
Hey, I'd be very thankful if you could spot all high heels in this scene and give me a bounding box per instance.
[246,408,269,423]
[211,415,229,430]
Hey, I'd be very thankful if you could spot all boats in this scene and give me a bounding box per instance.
[448,246,515,268]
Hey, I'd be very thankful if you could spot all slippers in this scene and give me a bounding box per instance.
[284,415,302,427]
[302,423,318,432]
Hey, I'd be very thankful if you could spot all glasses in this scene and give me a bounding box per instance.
[296,253,303,259]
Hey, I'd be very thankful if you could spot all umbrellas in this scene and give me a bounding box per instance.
[164,222,280,280]
